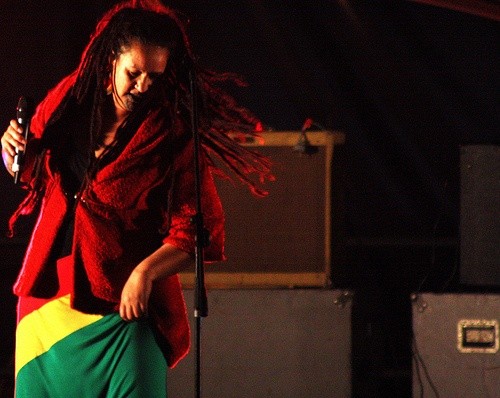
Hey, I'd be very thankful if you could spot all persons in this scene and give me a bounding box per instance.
[0,0,275,398]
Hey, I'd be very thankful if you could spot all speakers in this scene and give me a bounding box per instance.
[178,129,335,291]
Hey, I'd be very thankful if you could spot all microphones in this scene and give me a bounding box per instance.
[294,130,311,153]
[12,95,36,185]
